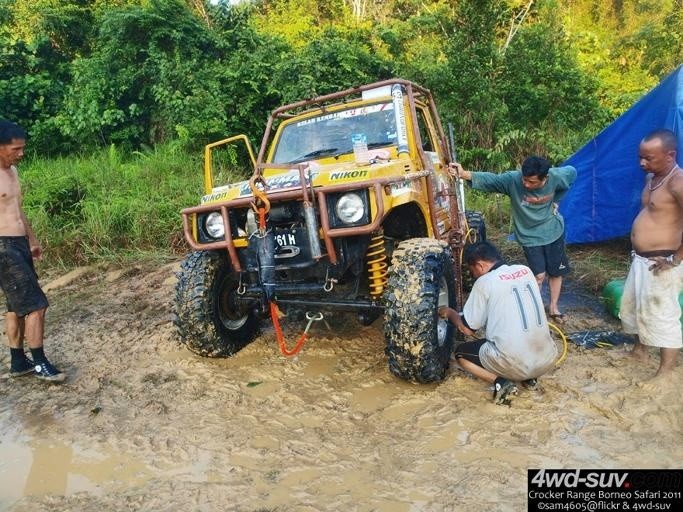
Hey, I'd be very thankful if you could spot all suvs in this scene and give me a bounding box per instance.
[169,77,487,384]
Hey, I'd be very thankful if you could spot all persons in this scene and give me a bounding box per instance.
[0,122,67,385]
[433,242,558,406]
[617,129,682,380]
[446,156,578,318]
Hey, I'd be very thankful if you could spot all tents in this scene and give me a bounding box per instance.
[506,65,682,244]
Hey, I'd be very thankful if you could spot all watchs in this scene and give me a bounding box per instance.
[666,255,677,266]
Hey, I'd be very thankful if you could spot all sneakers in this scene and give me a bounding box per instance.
[493,377,538,404]
[11,359,64,380]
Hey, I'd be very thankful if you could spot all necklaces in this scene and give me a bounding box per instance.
[647,164,677,191]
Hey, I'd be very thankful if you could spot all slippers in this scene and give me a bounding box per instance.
[550,314,566,323]
